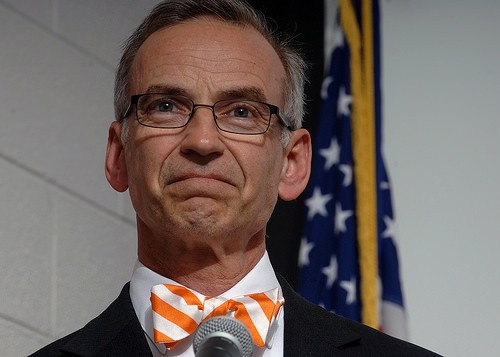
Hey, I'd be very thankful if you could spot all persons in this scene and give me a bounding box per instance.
[26,0,447,357]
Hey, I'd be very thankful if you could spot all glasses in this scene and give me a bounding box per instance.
[120,91,293,136]
[148,285,285,348]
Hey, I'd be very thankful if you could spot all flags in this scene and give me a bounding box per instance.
[293,0,408,342]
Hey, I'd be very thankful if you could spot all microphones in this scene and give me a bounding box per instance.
[193,314,254,357]
